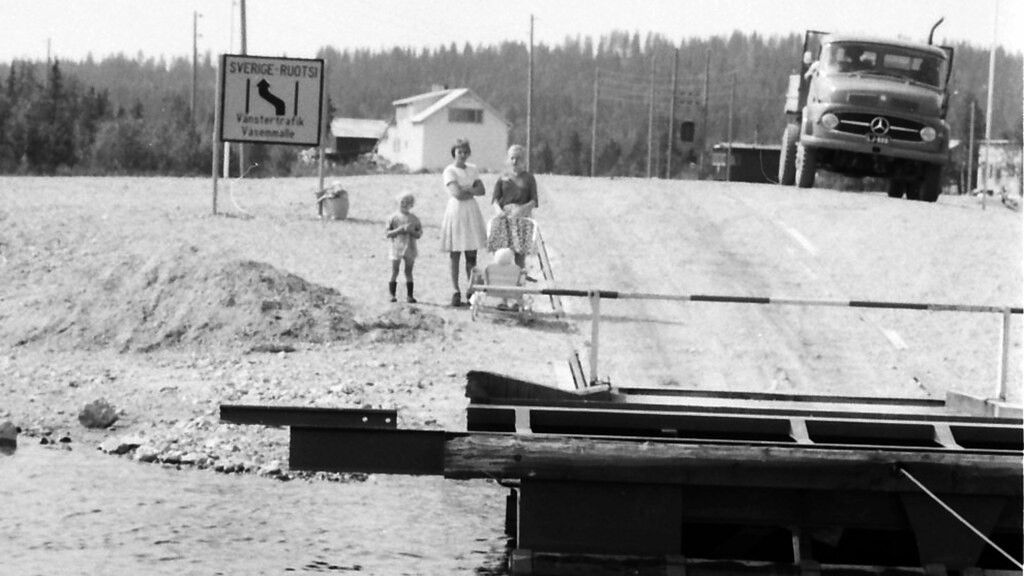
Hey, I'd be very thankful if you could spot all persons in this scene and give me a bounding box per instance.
[487,144,539,311]
[438,138,488,306]
[385,194,422,304]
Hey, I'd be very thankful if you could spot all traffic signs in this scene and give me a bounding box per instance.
[219,54,325,149]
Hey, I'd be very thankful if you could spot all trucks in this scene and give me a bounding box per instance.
[778,17,956,203]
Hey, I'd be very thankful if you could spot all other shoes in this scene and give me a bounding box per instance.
[452,293,461,307]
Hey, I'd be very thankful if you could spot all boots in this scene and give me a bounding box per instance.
[388,281,397,301]
[406,281,416,303]
